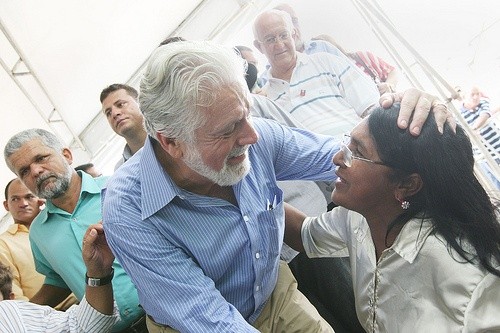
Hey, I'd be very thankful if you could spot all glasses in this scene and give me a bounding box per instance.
[339,134,388,168]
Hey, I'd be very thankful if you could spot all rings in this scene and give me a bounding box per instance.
[435,102,448,110]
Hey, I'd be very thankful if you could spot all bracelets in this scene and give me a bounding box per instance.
[85,267,116,287]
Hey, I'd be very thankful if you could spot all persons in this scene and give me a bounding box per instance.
[4,128,150,333]
[155,36,371,332]
[456,84,500,192]
[0,223,123,333]
[0,176,80,313]
[282,101,500,333]
[232,45,260,73]
[309,34,395,94]
[100,40,460,333]
[0,260,16,302]
[73,164,104,181]
[262,0,378,94]
[99,84,155,173]
[251,7,408,210]
